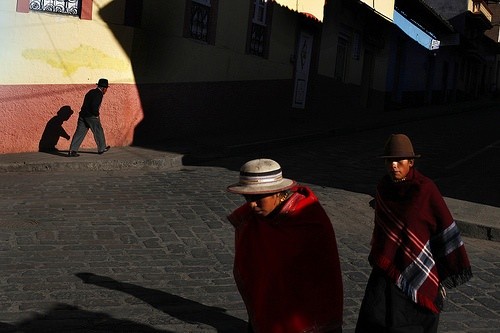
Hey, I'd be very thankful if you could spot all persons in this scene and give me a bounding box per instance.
[226,158,343,333]
[69,79,111,156]
[354,134,473,333]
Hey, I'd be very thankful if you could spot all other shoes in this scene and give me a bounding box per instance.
[98,147,109,154]
[68,151,80,157]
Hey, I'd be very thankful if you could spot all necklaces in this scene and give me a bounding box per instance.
[280,193,288,201]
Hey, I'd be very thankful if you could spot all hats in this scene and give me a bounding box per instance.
[97,79,110,87]
[377,134,416,158]
[226,158,296,194]
